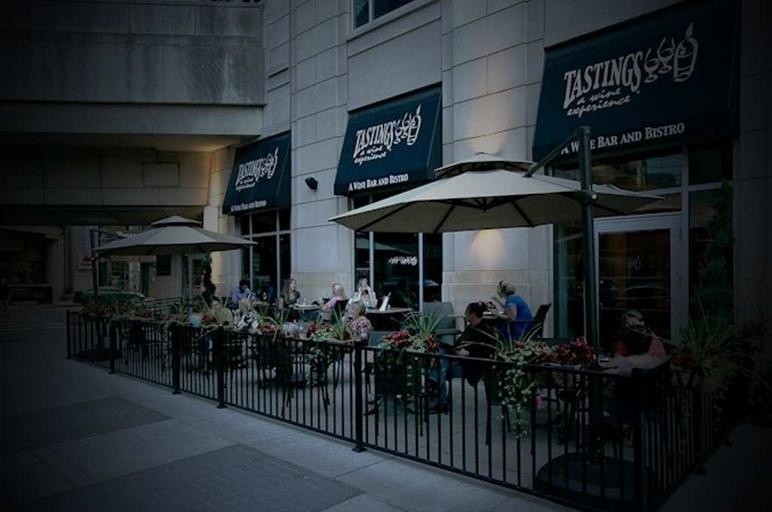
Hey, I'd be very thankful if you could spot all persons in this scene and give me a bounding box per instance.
[188,276,378,368]
[426,302,498,413]
[489,277,533,339]
[558,311,665,417]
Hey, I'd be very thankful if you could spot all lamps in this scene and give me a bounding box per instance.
[330,299,350,323]
[421,299,551,346]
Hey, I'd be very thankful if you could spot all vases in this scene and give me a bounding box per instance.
[77,298,192,376]
[375,308,445,410]
[501,334,598,438]
[663,315,769,428]
[205,308,348,407]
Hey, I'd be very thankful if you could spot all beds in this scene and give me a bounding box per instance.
[329,124,663,371]
[93,216,256,312]
